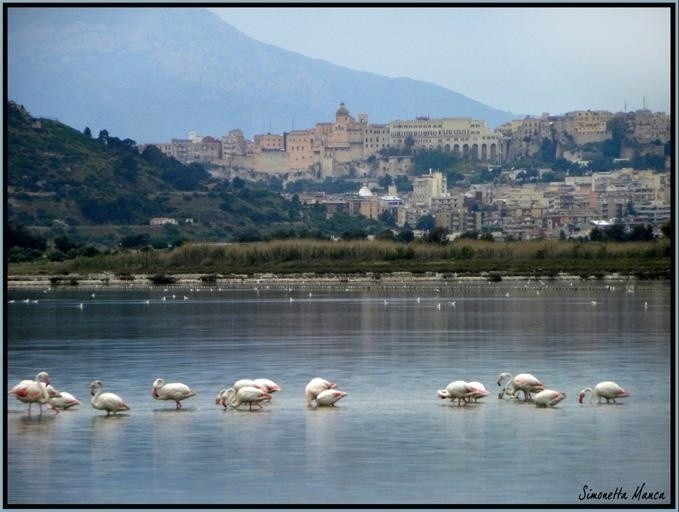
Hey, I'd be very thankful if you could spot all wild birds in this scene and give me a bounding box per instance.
[437,381,491,406]
[8,372,81,421]
[152,378,195,410]
[496,372,567,409]
[579,381,630,404]
[89,380,130,416]
[215,379,282,412]
[305,377,348,409]
